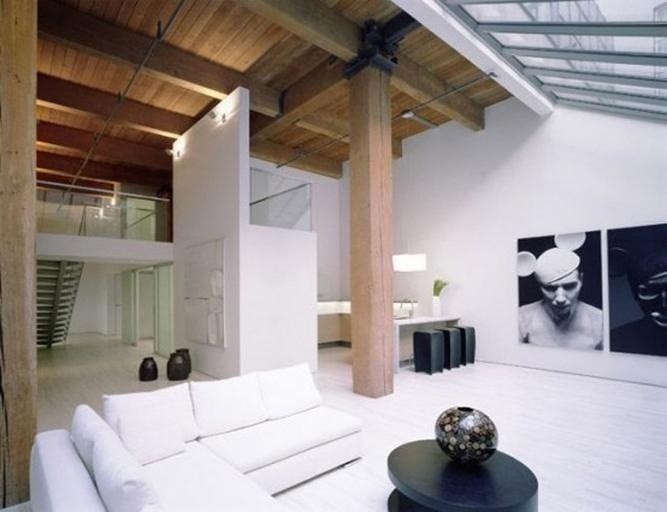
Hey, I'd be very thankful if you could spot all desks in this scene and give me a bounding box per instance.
[393,318,459,374]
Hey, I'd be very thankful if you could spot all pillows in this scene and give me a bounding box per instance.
[93,431,183,512]
[70,403,124,478]
[97,383,197,466]
[190,373,271,439]
[252,363,321,420]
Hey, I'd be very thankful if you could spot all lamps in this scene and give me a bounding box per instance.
[393,252,427,319]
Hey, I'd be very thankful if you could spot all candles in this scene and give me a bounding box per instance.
[431,278,449,320]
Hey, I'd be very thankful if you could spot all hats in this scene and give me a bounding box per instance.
[628,255,667,283]
[535,248,580,284]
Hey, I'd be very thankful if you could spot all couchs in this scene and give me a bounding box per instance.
[189,364,363,496]
[31,383,284,512]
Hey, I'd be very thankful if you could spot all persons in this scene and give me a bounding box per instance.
[610,253,667,356]
[519,252,603,351]
